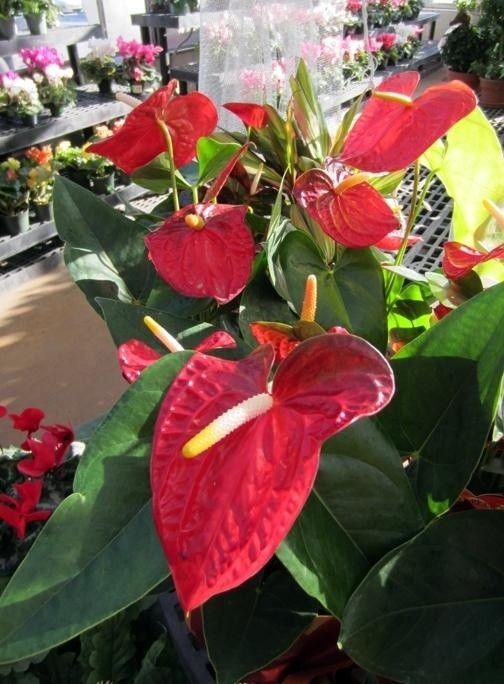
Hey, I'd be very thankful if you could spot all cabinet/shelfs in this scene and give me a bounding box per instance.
[129,10,445,119]
[0,24,163,269]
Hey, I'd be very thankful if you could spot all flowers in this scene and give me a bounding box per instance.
[202,0,424,107]
[0,66,504,683]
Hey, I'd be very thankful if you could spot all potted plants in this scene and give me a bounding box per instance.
[441,0,504,108]
